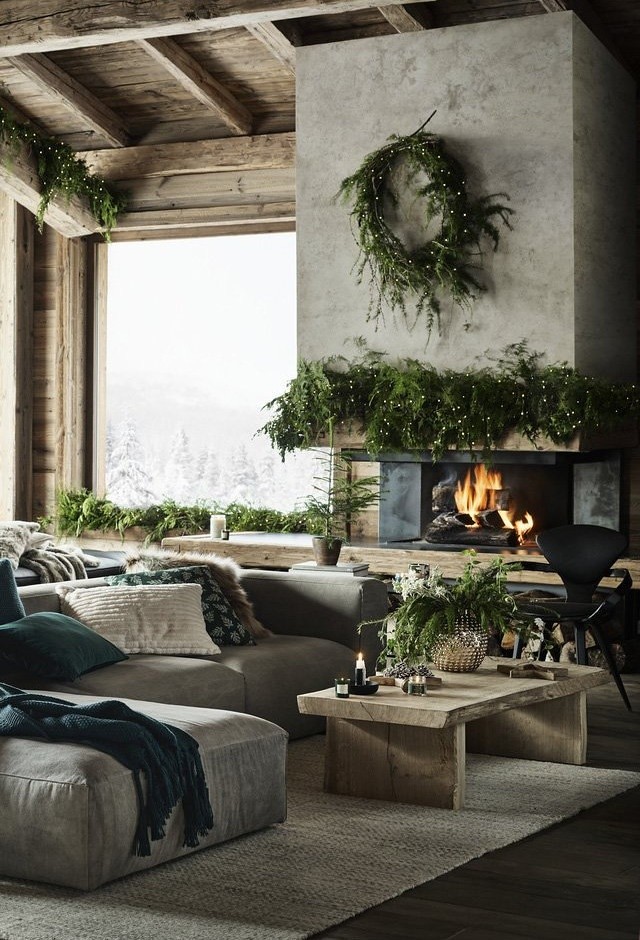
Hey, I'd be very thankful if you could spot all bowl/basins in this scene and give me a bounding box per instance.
[349,680,379,694]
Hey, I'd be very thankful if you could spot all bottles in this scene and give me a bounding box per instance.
[408,676,427,696]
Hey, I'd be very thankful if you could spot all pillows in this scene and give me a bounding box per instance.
[0,520,266,679]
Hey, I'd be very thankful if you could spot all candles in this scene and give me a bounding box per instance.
[355,653,366,685]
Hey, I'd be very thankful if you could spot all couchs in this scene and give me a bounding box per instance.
[0,568,389,893]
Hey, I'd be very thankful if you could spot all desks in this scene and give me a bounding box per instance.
[296,652,612,810]
[0,546,146,584]
[159,525,640,609]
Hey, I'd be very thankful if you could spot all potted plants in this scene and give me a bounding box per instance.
[356,544,560,674]
[298,416,387,567]
[0,105,122,245]
[251,335,640,468]
[36,485,335,558]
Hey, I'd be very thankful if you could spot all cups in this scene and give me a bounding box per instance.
[221,530,230,540]
[335,678,351,698]
[211,515,226,537]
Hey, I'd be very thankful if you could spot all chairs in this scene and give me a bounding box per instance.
[479,524,637,714]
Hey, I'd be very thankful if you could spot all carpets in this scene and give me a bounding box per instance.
[0,726,640,940]
